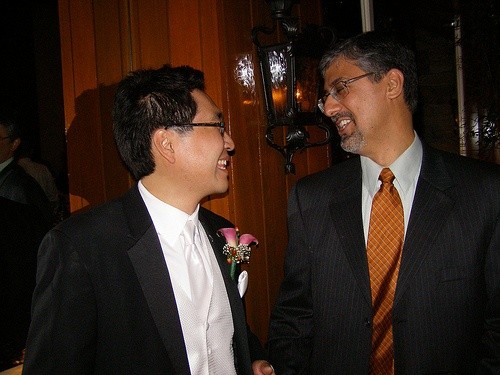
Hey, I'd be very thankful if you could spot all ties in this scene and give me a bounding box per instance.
[366,168,405,375]
[181,220,209,327]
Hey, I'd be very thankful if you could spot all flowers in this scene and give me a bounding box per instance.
[219,224,259,290]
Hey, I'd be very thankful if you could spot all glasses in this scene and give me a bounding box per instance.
[318,69,389,114]
[166,112,225,136]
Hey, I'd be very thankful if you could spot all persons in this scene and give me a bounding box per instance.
[22,65,276,375]
[267,31,500,375]
[0,113,49,372]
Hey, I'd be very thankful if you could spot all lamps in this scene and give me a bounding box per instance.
[234,0,334,174]
